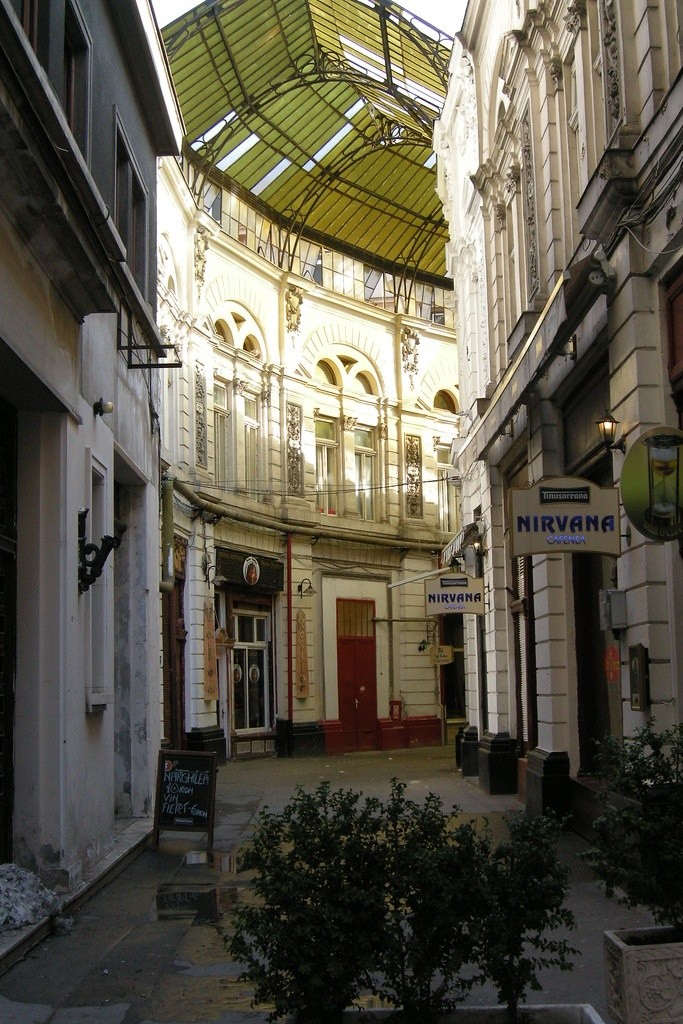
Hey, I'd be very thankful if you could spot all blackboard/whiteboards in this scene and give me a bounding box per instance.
[154,750,217,833]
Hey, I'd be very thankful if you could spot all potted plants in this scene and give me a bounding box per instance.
[217,782,604,1024]
[575,716,683,1024]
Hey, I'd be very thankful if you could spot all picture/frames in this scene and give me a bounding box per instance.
[629,643,649,711]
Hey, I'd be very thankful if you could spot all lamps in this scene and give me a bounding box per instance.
[299,578,317,599]
[205,566,227,587]
[594,410,627,456]
